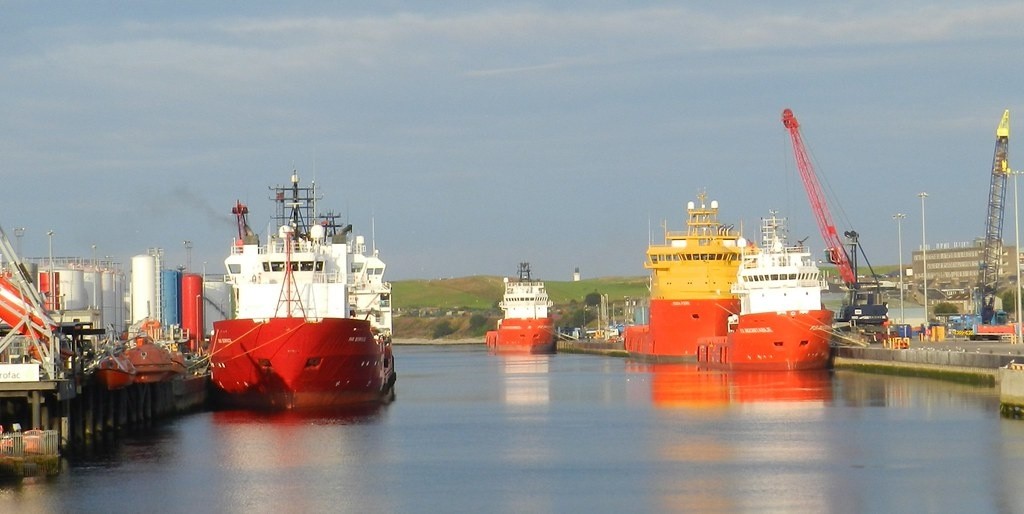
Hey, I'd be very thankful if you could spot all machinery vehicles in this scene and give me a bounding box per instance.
[780,107,890,343]
[953,108,1016,341]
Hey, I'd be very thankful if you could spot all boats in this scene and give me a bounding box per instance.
[209,167,398,412]
[624,186,759,356]
[485,259,554,353]
[697,207,835,371]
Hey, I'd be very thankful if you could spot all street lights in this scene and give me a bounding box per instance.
[916,192,930,329]
[892,213,909,323]
